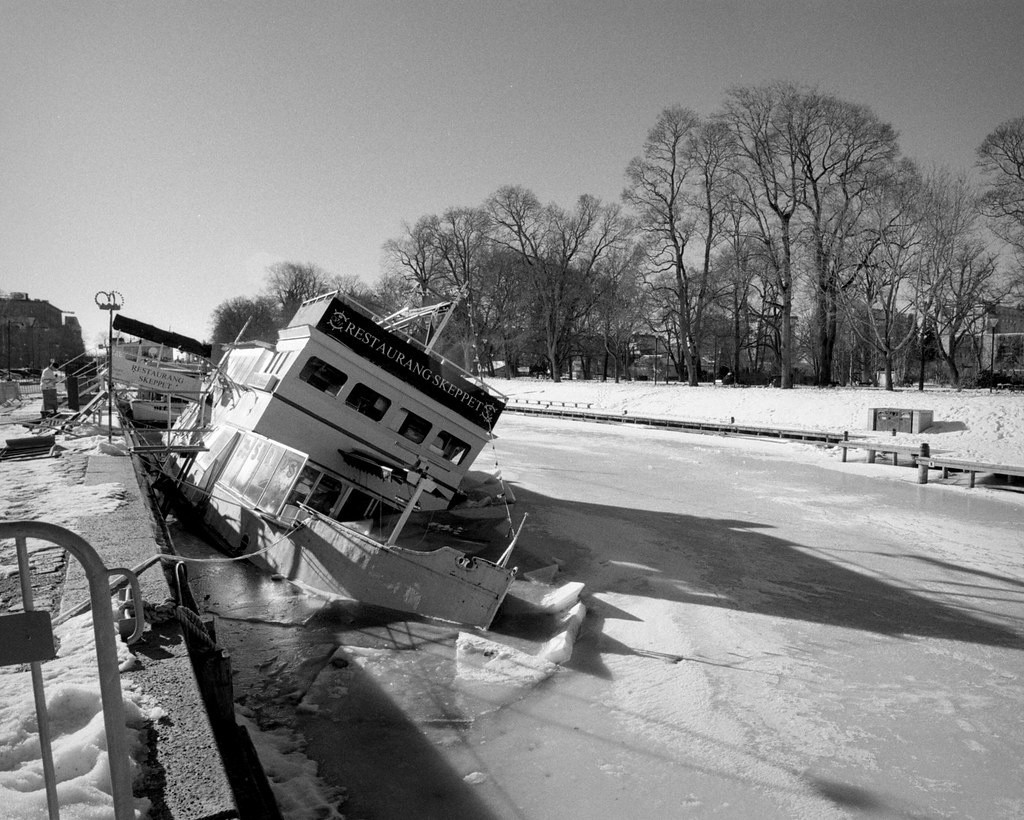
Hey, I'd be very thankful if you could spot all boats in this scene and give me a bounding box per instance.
[156,279,530,634]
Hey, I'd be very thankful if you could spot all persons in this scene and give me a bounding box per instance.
[40,362,59,417]
